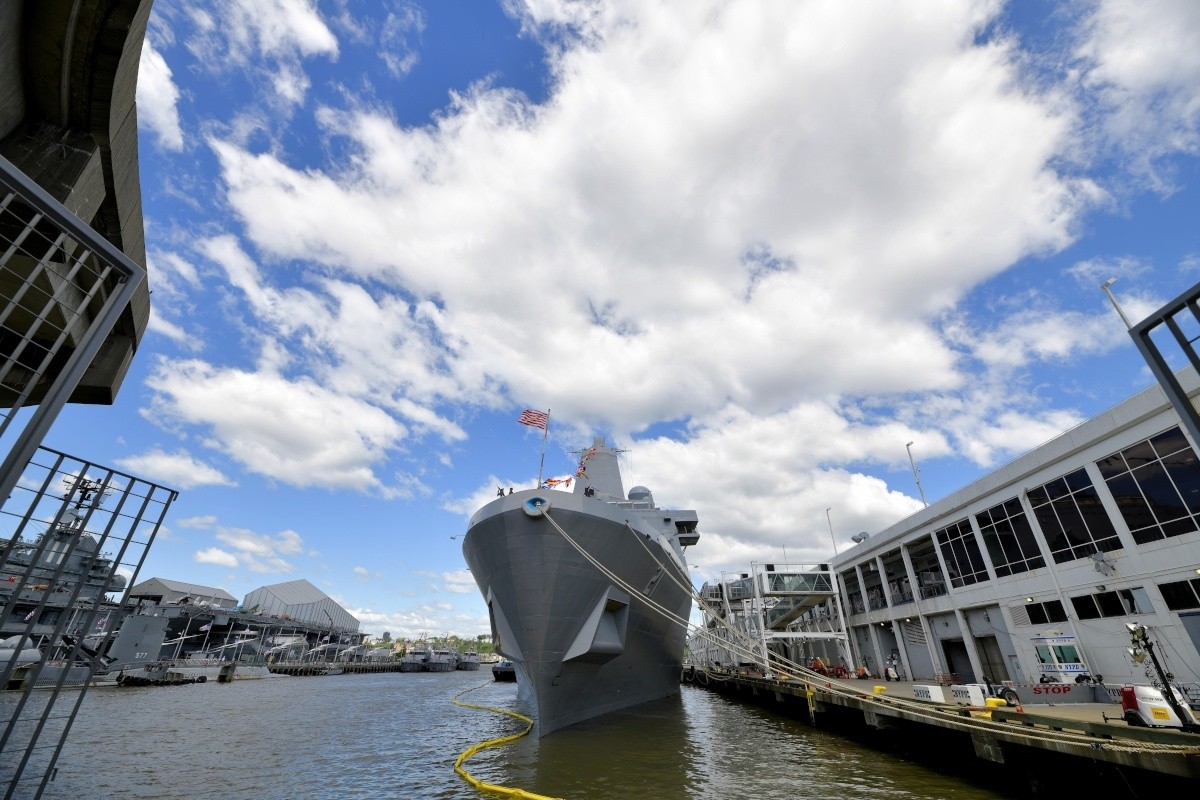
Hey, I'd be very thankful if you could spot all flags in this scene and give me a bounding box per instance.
[544,478,560,486]
[518,409,548,430]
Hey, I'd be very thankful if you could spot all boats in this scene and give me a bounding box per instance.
[491,659,515,682]
[462,433,700,739]
[205,609,480,675]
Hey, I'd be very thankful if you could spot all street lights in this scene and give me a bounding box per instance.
[826,507,837,554]
[906,441,928,508]
[1100,277,1133,330]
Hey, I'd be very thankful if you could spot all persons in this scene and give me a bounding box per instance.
[806,656,823,673]
[1039,674,1049,684]
